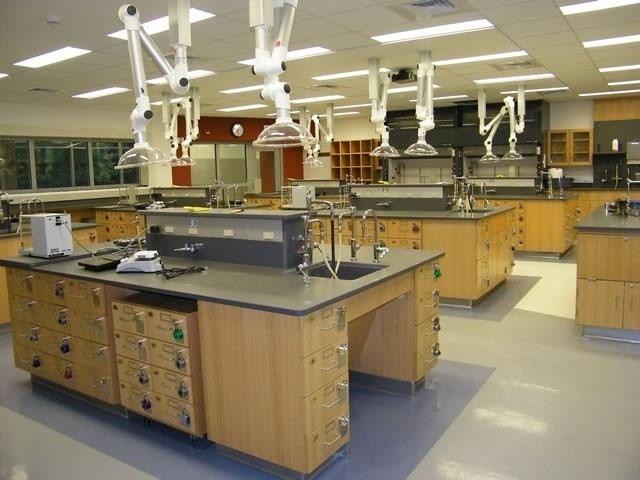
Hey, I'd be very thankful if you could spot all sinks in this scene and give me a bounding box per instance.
[450,207,493,214]
[284,258,391,281]
[204,200,245,208]
[0,227,32,235]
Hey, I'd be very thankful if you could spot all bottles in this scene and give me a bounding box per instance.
[611,139,619,151]
[604,198,628,216]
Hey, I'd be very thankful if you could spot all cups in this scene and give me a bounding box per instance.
[281,186,292,208]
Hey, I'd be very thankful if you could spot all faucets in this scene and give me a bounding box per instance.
[20,197,45,212]
[206,179,240,205]
[449,174,495,208]
[295,190,387,264]
[539,171,550,189]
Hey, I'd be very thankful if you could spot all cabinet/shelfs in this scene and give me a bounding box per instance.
[545,124,594,167]
[457,97,550,159]
